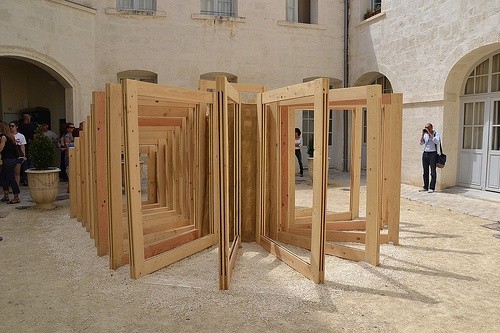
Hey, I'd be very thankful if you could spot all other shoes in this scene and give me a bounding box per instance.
[418,188,427,192]
[23,179,28,186]
[428,189,434,193]
[300,174,303,177]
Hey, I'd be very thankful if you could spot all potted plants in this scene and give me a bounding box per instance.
[364,5,381,19]
[24,124,61,209]
[307,135,331,185]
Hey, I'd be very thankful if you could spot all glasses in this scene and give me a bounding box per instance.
[69,126,75,129]
[9,125,16,128]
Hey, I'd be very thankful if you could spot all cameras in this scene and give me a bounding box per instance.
[423,129,428,133]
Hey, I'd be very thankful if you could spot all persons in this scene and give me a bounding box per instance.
[0,112,84,204]
[418,123,440,193]
[295,128,304,177]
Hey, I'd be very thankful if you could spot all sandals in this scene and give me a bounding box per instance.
[0,196,9,202]
[6,199,20,204]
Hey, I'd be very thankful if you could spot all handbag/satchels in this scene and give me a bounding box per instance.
[436,154,447,168]
